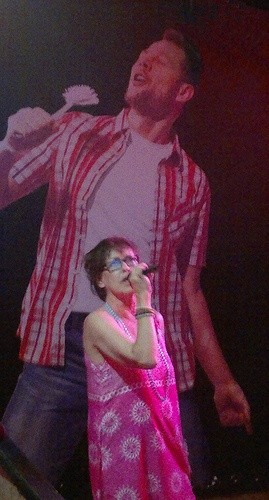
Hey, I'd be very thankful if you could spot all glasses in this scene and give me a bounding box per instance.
[101,255,138,272]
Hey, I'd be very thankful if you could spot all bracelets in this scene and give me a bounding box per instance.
[135,306,154,319]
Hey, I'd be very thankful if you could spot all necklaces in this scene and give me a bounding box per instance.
[104,301,170,400]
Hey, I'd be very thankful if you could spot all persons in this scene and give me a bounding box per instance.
[81,236,196,500]
[0,30,249,500]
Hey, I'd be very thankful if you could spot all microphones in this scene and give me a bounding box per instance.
[142,266,162,276]
